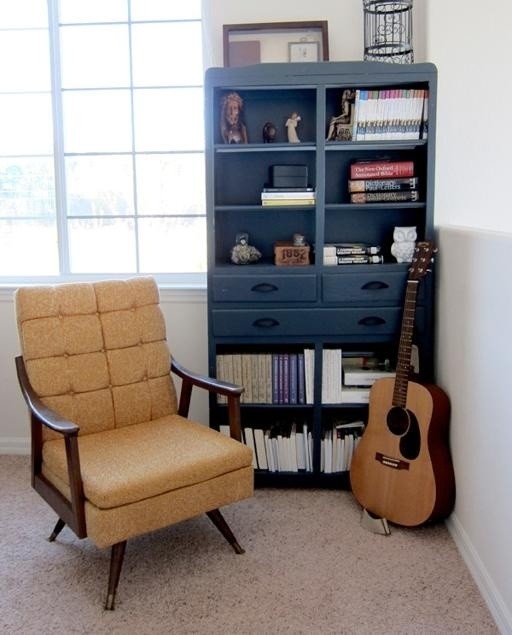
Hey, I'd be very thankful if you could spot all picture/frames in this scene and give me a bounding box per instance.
[221,19,328,66]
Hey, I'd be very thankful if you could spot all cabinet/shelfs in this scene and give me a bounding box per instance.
[202,62,438,492]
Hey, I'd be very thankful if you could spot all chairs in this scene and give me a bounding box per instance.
[12,274,259,611]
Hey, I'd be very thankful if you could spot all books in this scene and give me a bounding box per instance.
[260,187,316,207]
[216,347,396,475]
[351,89,429,142]
[347,160,419,204]
[323,243,384,267]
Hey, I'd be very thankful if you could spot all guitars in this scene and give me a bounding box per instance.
[350,240,456,528]
